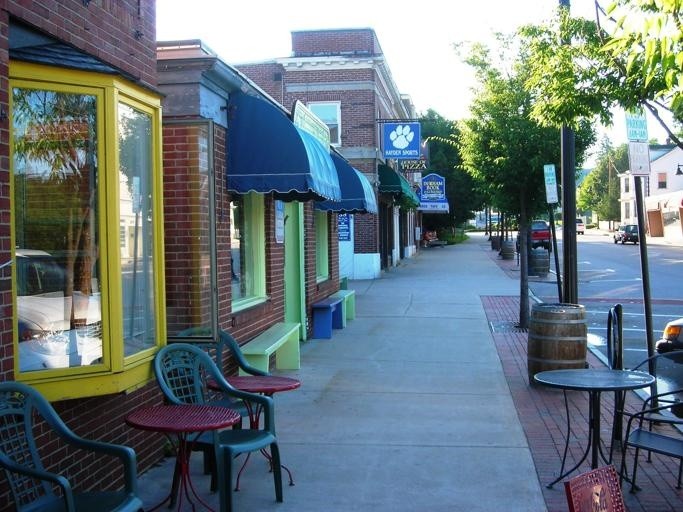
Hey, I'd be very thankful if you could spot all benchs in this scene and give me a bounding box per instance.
[237,322,300,379]
[312,288,359,340]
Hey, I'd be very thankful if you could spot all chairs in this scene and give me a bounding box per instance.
[616,350,683,495]
[155,328,283,511]
[0,382,141,512]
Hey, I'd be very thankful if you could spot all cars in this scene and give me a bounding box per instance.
[15,248,103,358]
[516,220,552,253]
[575,218,585,235]
[612,225,638,245]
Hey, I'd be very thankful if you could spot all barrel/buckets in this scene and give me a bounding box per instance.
[501,240,515,260]
[528,302,587,390]
[529,248,550,278]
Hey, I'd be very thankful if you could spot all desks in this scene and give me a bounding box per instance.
[206,373,300,494]
[123,404,243,512]
[533,368,656,495]
